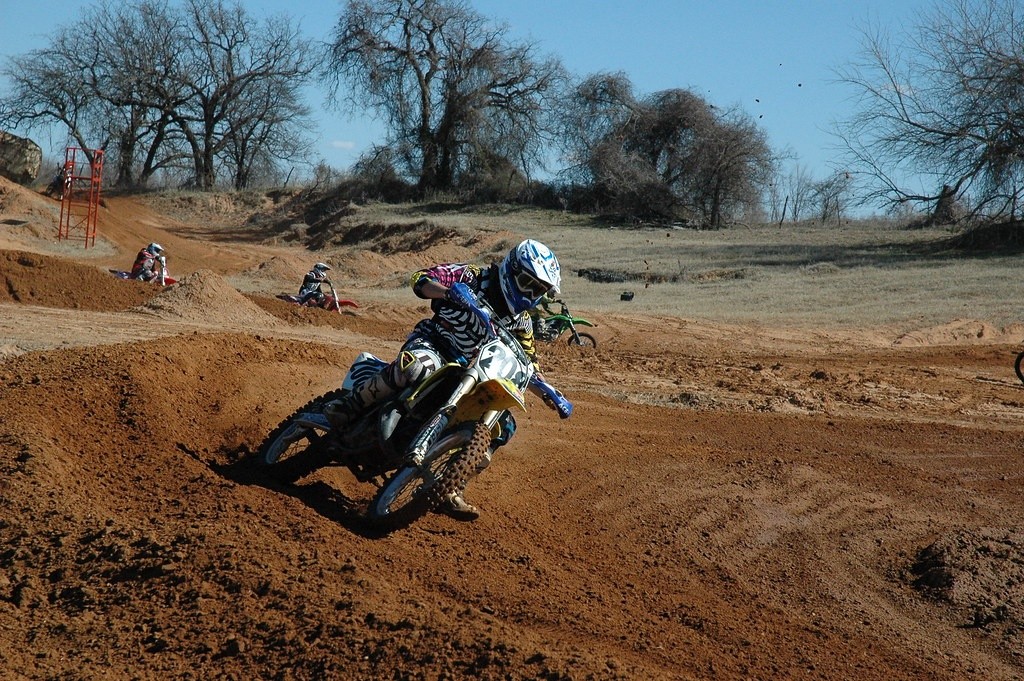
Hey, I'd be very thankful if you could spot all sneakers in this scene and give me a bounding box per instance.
[430,495,479,521]
[323,397,352,427]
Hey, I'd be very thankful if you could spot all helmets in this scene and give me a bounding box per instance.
[147,243,164,257]
[314,263,331,277]
[499,239,561,315]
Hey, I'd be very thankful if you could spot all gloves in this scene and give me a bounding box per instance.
[443,281,478,310]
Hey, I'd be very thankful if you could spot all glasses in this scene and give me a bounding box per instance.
[515,269,548,300]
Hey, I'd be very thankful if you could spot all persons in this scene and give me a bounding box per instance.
[323,239,561,521]
[131,243,164,284]
[299,262,331,308]
[54,160,72,191]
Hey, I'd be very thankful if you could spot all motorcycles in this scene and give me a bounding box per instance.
[108,254,178,289]
[254,289,575,536]
[526,298,597,349]
[46,169,74,202]
[275,279,358,317]
